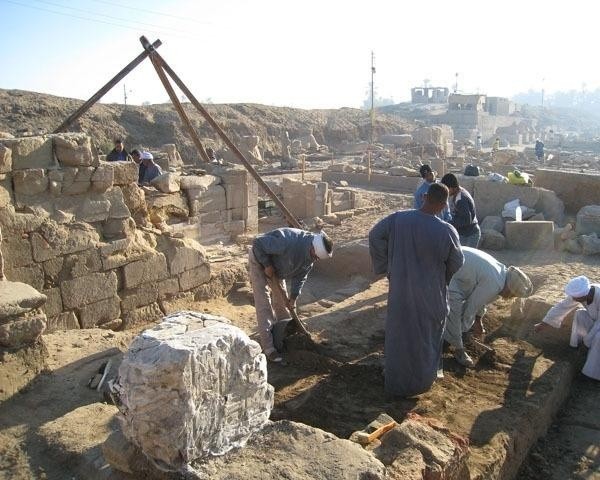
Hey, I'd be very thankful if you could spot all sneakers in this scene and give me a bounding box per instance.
[268,352,282,362]
[455,350,473,365]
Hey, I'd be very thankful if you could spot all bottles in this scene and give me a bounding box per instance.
[516,205,522,222]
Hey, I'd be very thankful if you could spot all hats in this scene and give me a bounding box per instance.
[441,173,457,187]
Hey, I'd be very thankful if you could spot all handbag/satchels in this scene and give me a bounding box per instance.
[465,165,479,175]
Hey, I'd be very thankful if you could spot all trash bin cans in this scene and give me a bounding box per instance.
[493,136,500,150]
[477,135,482,150]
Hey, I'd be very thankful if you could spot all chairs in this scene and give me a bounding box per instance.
[258,201,271,217]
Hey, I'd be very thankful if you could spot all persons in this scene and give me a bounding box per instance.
[534,140,546,164]
[368,183,466,400]
[443,244,532,368]
[493,138,501,153]
[141,151,162,185]
[413,164,452,222]
[245,227,333,363]
[533,275,600,381]
[106,139,133,162]
[477,135,482,151]
[130,150,146,184]
[441,173,481,248]
